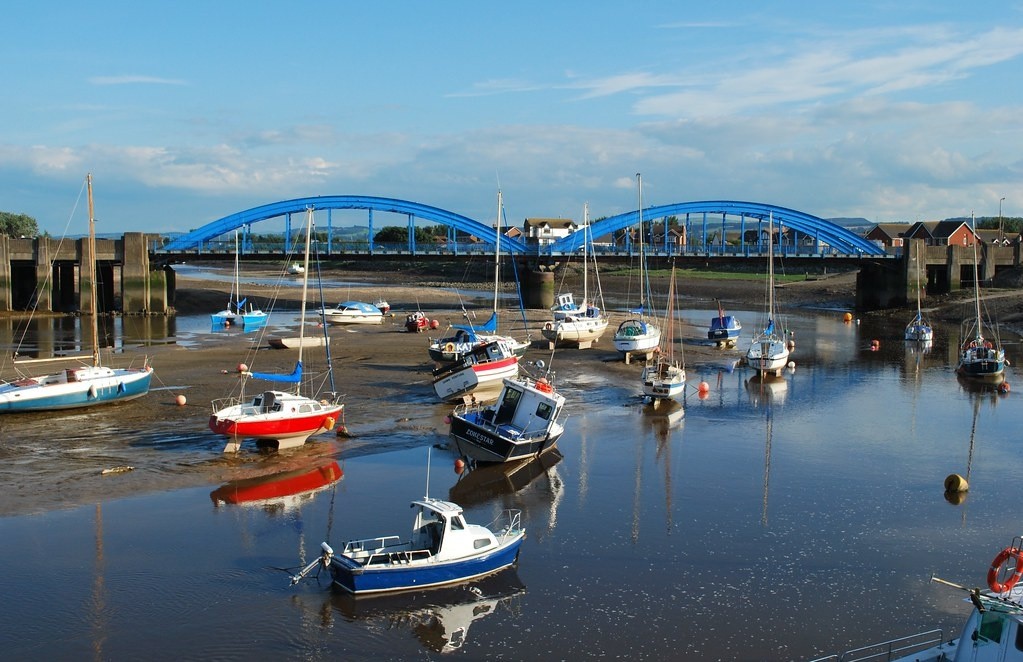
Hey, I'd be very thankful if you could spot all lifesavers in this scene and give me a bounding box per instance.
[986,547,1023,593]
[546,323,551,329]
[564,304,571,309]
[445,342,454,351]
[536,381,552,392]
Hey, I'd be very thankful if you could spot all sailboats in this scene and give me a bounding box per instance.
[541,203,611,350]
[431,174,531,359]
[0,174,154,415]
[906,242,933,342]
[746,209,796,370]
[209,202,345,454]
[610,171,662,354]
[209,228,270,332]
[641,256,687,398]
[955,214,1010,385]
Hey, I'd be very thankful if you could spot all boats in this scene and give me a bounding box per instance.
[291,447,527,604]
[443,334,570,467]
[708,298,743,342]
[404,296,439,333]
[267,336,332,349]
[287,261,304,275]
[208,460,344,511]
[320,303,385,326]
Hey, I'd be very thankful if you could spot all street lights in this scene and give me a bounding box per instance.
[998,198,1005,247]
